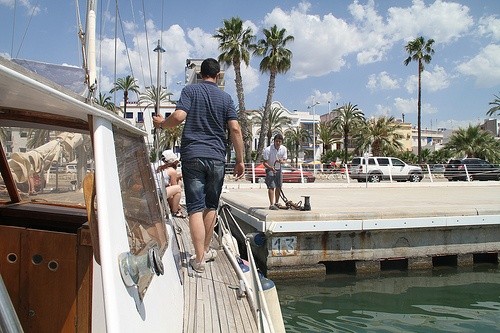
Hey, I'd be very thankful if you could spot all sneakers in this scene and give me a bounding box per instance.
[204,249,217,261]
[189,255,206,272]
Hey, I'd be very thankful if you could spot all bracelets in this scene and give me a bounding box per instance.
[160,121,163,129]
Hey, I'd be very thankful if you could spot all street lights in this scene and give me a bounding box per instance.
[308,95,320,176]
[153,39,166,117]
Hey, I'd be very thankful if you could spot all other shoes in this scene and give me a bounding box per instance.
[275,203,282,207]
[270,205,279,210]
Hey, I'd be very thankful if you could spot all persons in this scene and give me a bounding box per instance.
[155,150,186,218]
[263,134,288,209]
[153,58,245,273]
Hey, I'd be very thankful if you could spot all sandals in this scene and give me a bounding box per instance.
[172,211,186,218]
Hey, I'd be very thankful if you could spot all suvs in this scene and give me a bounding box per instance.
[443,158,500,181]
[349,157,424,183]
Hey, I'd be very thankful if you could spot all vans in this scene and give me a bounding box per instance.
[66,165,90,173]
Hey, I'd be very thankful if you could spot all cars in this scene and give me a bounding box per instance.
[245,161,316,183]
